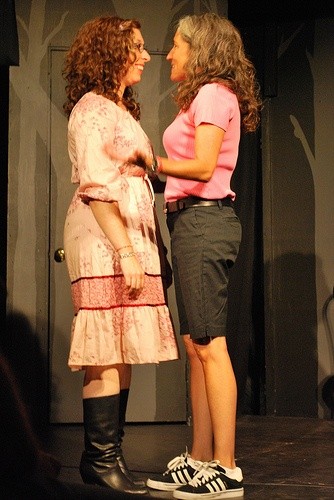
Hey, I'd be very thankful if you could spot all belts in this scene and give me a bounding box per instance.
[162,196,236,214]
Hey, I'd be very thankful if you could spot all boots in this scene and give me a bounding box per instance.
[117,388,147,487]
[79,394,150,496]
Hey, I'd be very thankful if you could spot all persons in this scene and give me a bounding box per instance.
[64,16,179,493]
[122,14,263,500]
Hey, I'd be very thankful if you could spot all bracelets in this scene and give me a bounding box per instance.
[118,252,135,259]
[155,157,162,173]
[116,244,134,251]
[150,160,159,171]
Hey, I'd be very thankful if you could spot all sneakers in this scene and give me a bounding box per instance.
[173,460,244,499]
[147,446,203,491]
